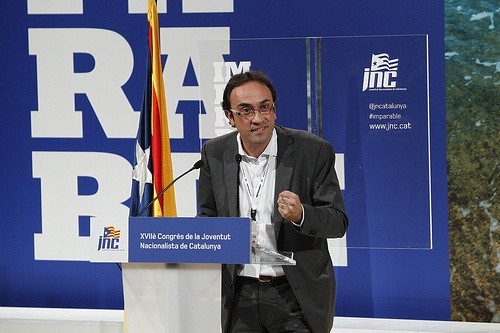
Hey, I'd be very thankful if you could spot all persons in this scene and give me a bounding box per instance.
[195,73,349,333]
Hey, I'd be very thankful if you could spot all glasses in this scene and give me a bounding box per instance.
[228,102,275,120]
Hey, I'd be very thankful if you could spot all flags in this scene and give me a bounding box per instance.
[130,0,177,217]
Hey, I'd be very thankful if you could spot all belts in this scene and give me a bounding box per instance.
[235,275,287,282]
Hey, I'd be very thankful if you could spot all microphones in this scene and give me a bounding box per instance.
[235,154,242,216]
[138,160,204,217]
